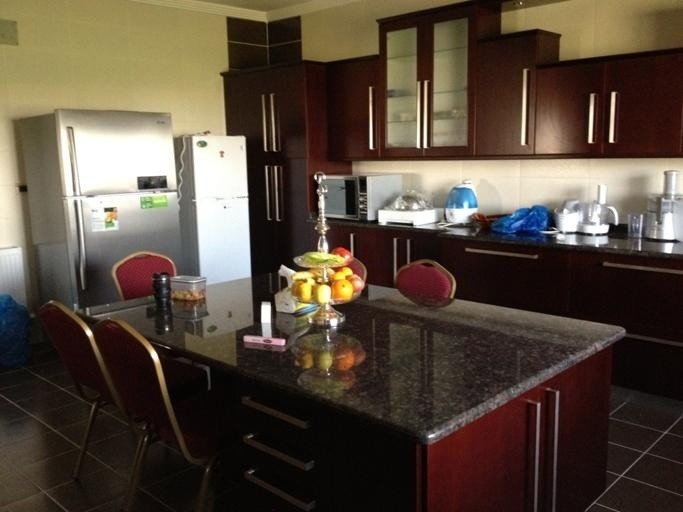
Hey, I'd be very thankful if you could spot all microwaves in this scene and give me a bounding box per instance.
[325,173,405,221]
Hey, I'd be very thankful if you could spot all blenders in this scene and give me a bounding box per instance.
[552,184,620,234]
[642,170,682,242]
[553,231,610,250]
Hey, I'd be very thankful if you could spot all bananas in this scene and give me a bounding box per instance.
[303,252,345,265]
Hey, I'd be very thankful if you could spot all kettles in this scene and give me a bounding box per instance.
[445,179,478,224]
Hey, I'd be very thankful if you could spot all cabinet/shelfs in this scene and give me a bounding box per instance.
[223,60,332,158]
[422,348,612,512]
[325,224,376,285]
[475,29,560,160]
[536,47,683,159]
[439,234,575,318]
[252,261,307,324]
[375,227,439,288]
[577,245,683,400]
[377,0,500,160]
[248,158,333,259]
[326,56,382,161]
[221,380,422,512]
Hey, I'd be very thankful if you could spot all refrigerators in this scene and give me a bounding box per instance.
[18,108,183,307]
[186,275,254,339]
[77,295,187,350]
[172,134,251,282]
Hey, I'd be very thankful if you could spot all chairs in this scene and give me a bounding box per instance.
[308,247,368,289]
[37,300,144,512]
[395,261,457,298]
[93,319,221,512]
[111,252,177,301]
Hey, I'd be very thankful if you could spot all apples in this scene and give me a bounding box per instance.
[332,247,352,264]
[354,350,366,366]
[301,352,332,370]
[346,274,365,294]
[291,267,334,305]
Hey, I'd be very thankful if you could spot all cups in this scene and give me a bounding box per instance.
[154,307,174,334]
[152,272,171,306]
[627,212,644,238]
